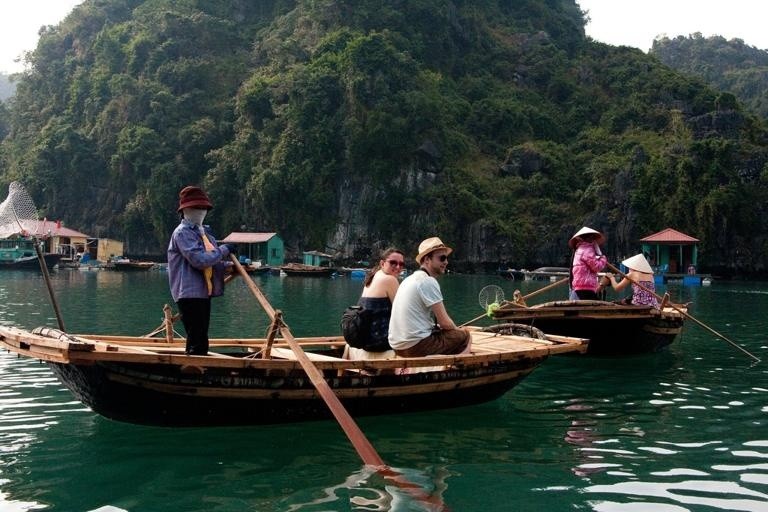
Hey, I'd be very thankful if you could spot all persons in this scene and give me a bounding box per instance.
[357,247,404,353]
[688,264,696,276]
[166,185,237,354]
[387,236,472,355]
[568,225,657,309]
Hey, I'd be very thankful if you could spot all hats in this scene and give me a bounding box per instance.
[568,226,606,250]
[415,236,454,266]
[177,185,214,214]
[621,253,654,274]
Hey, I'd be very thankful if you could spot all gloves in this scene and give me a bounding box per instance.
[232,261,249,273]
[224,243,237,255]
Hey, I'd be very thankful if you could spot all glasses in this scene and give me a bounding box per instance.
[428,255,449,263]
[382,259,405,268]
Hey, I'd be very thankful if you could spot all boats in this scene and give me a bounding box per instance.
[0,314,591,428]
[213,227,409,281]
[0,238,154,274]
[494,229,713,290]
[483,287,689,354]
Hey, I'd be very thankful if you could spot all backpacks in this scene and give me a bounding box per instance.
[341,305,390,349]
